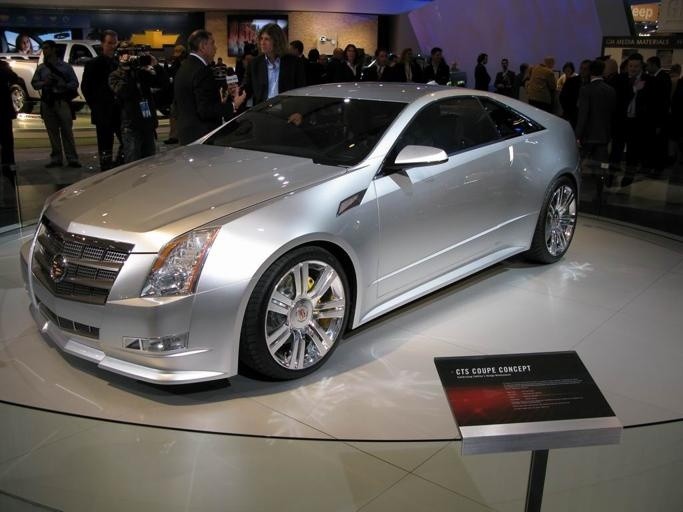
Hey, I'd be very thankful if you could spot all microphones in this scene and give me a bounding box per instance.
[226,66,238,85]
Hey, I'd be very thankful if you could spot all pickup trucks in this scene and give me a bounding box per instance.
[1,36,103,111]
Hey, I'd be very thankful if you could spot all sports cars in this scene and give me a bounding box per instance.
[15,78,583,391]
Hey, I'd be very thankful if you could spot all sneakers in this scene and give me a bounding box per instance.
[71,161,82,168]
[44,160,64,168]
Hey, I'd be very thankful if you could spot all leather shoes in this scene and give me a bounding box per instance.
[604,172,683,187]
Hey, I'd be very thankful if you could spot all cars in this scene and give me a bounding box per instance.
[117,46,175,115]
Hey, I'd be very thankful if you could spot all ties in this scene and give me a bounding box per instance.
[631,76,637,87]
